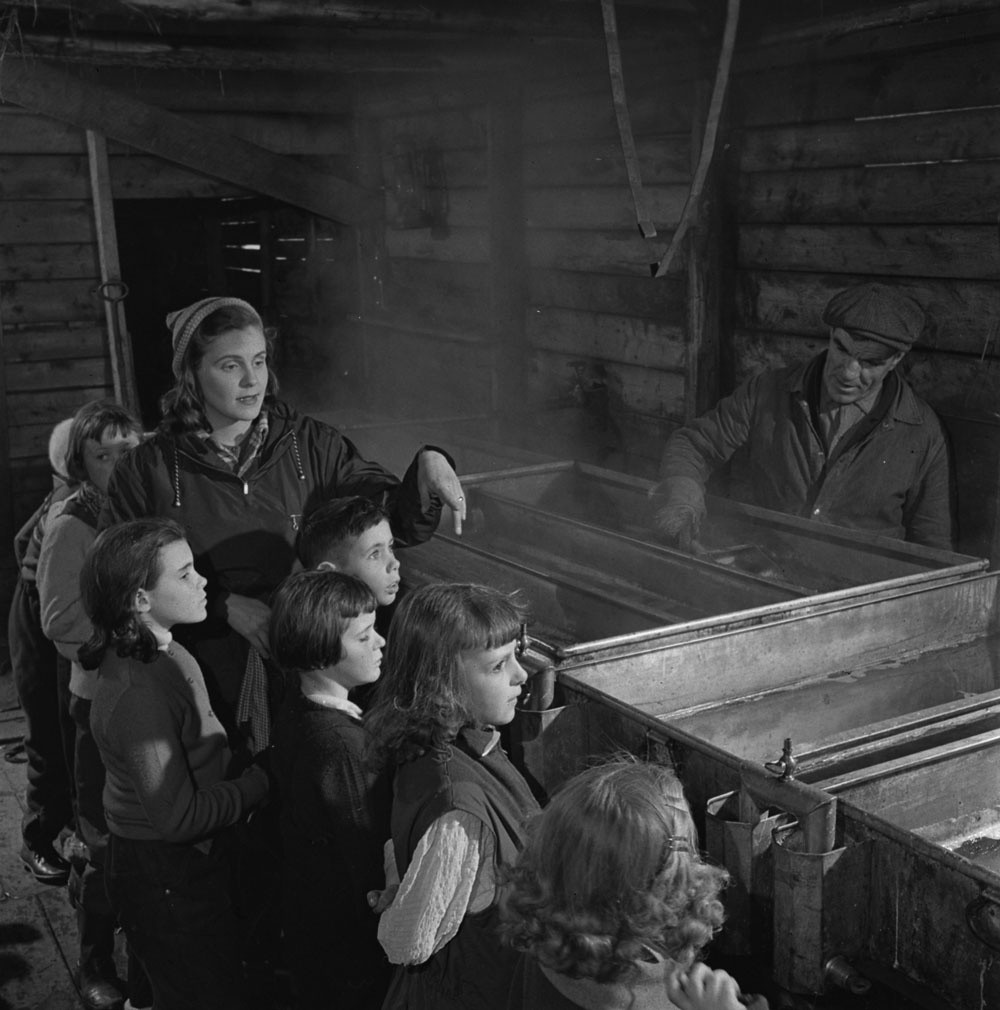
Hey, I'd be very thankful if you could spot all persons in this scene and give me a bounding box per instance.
[368,584,541,1010]
[650,283,953,551]
[8,298,465,1010]
[500,751,747,1010]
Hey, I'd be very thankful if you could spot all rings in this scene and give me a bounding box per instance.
[458,497,464,502]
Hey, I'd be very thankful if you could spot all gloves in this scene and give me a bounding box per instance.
[647,476,707,553]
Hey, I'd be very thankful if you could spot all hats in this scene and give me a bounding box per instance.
[49,418,73,480]
[822,283,926,354]
[166,297,264,379]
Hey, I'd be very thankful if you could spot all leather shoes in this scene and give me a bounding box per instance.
[73,959,127,1010]
[18,840,70,885]
